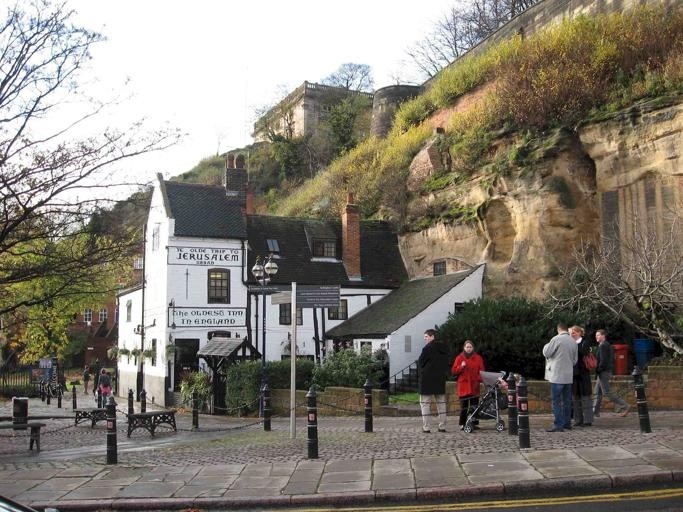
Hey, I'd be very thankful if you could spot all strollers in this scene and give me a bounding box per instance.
[463,370,508,433]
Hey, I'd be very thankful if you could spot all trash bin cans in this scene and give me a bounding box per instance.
[12,397,29,430]
[632,338,655,370]
[611,344,631,375]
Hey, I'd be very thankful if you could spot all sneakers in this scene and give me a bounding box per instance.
[596,414,600,417]
[619,405,632,417]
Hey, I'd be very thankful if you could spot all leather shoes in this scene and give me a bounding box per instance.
[562,425,572,429]
[545,427,563,432]
[439,429,446,432]
[422,428,430,433]
[574,423,592,427]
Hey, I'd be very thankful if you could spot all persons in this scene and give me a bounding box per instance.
[450,340,485,431]
[98,370,113,404]
[417,328,450,433]
[83,365,91,395]
[92,361,101,396]
[593,329,631,418]
[542,322,578,433]
[566,325,595,427]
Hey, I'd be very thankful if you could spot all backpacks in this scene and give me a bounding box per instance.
[583,351,598,372]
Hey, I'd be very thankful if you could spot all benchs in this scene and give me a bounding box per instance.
[74,409,179,437]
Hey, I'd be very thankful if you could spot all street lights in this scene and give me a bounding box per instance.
[251,253,279,430]
